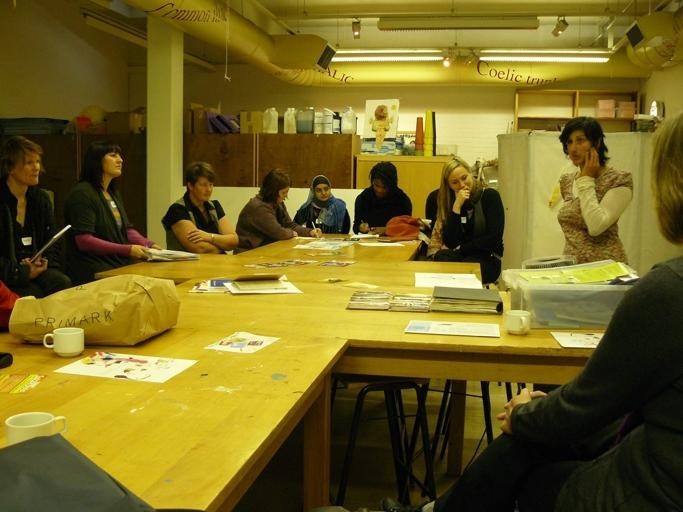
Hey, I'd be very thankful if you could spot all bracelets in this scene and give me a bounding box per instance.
[210,233,215,245]
[12,265,20,286]
[233,166,323,257]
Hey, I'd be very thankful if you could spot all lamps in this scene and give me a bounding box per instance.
[330,48,458,65]
[463,48,611,65]
[551,16,569,37]
[351,17,361,40]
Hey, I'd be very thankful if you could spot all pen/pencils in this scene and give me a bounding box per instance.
[312,221,320,239]
[361,219,365,226]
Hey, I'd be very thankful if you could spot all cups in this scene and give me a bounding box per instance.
[414,111,434,157]
[395,149,402,155]
[504,309,532,334]
[43,327,85,357]
[4,411,67,445]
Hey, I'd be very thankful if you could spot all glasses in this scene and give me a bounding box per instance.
[565,136,586,145]
[316,186,332,193]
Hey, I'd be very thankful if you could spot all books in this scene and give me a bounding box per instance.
[188,271,305,296]
[518,260,640,329]
[143,244,200,264]
[595,97,617,119]
[28,223,72,265]
[209,112,242,136]
[345,288,432,315]
[0,117,70,138]
[616,99,637,119]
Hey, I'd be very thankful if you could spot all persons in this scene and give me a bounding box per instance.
[555,115,634,267]
[433,155,506,284]
[378,111,683,512]
[159,160,240,256]
[62,141,162,276]
[0,135,72,299]
[352,160,413,236]
[292,174,351,236]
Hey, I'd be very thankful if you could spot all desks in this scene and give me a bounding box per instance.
[169,277,614,386]
[234,233,422,264]
[0,336,349,510]
[93,253,482,286]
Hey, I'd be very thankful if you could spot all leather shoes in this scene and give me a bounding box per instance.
[379,494,426,512]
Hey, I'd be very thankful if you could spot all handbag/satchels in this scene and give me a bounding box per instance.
[9,275,180,346]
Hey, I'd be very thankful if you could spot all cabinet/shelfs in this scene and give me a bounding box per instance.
[75,133,254,225]
[495,129,646,275]
[512,88,640,131]
[254,133,358,195]
[354,154,453,222]
